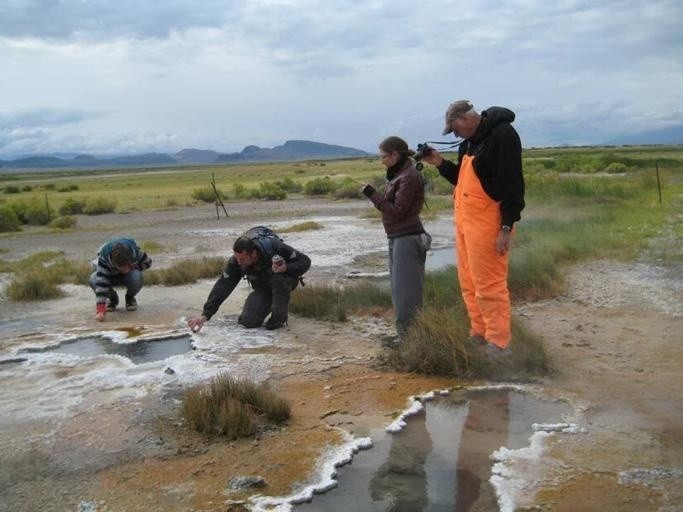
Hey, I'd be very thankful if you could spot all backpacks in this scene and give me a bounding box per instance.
[241,225,284,273]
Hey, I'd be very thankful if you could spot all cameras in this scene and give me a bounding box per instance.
[272,255,284,267]
[413,143,431,161]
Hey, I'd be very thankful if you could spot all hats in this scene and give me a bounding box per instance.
[440,99,473,136]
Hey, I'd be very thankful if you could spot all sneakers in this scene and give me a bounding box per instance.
[265,313,287,330]
[124,295,137,312]
[105,290,118,312]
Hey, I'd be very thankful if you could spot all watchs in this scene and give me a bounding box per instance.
[500,225,512,233]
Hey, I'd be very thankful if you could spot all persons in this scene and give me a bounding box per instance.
[188,226,310,332]
[90,238,152,321]
[360,136,431,344]
[421,100,525,359]
